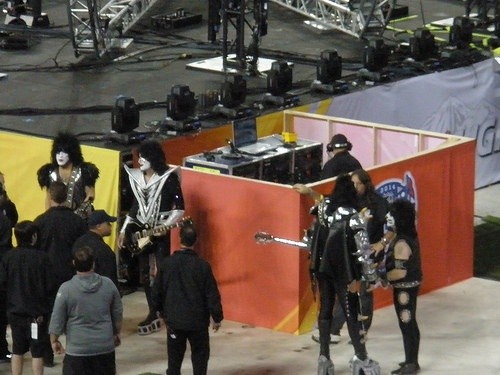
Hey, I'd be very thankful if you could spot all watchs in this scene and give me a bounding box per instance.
[381,237,389,244]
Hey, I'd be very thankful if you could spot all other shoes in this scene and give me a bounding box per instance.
[0,350,12,360]
[349,355,380,375]
[137,314,161,335]
[391,362,420,375]
[317,356,335,375]
[312,333,341,343]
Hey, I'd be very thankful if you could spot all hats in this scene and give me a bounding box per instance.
[87,210,117,225]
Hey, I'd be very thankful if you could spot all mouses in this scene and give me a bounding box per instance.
[284,144,296,148]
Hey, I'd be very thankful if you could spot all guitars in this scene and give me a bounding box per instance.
[124,215,193,255]
[74,196,95,215]
[254,231,311,249]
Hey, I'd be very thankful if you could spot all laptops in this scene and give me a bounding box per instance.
[232,117,278,155]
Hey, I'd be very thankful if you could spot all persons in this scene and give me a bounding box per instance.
[116,140,185,335]
[0,220,57,375]
[381,198,423,375]
[73,209,122,300]
[47,245,123,375]
[322,133,363,180]
[33,181,88,367]
[0,171,18,261]
[36,131,99,232]
[292,168,390,345]
[306,175,383,375]
[152,225,224,375]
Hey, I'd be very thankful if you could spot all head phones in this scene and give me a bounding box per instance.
[327,142,352,152]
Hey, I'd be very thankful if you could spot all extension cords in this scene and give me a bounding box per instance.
[180,54,192,59]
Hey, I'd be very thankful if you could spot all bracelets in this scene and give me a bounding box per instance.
[379,241,386,247]
